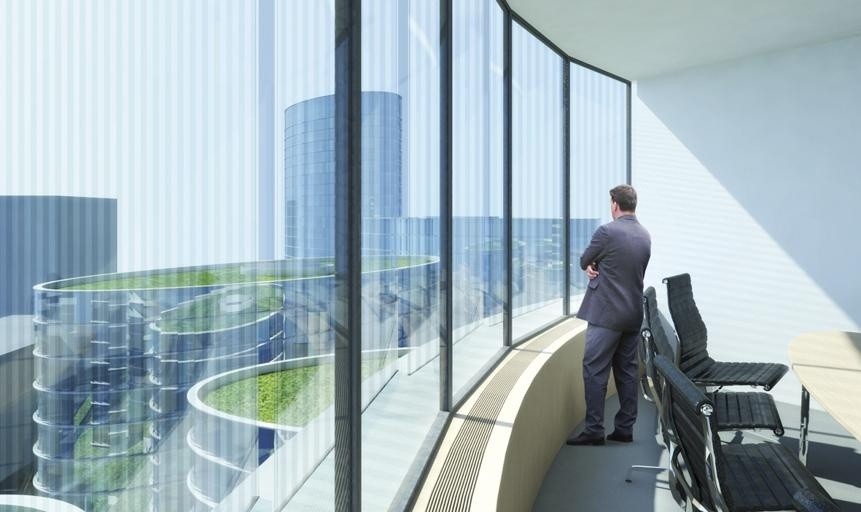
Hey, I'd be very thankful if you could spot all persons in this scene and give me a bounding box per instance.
[565,185,653,445]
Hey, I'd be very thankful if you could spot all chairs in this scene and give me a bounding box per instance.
[640,319,784,455]
[794,487,844,512]
[663,274,788,392]
[654,355,842,512]
[642,286,678,367]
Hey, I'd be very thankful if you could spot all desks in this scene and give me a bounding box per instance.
[787,329,861,466]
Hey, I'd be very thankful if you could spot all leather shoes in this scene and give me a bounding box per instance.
[607,431,632,442]
[567,432,605,445]
